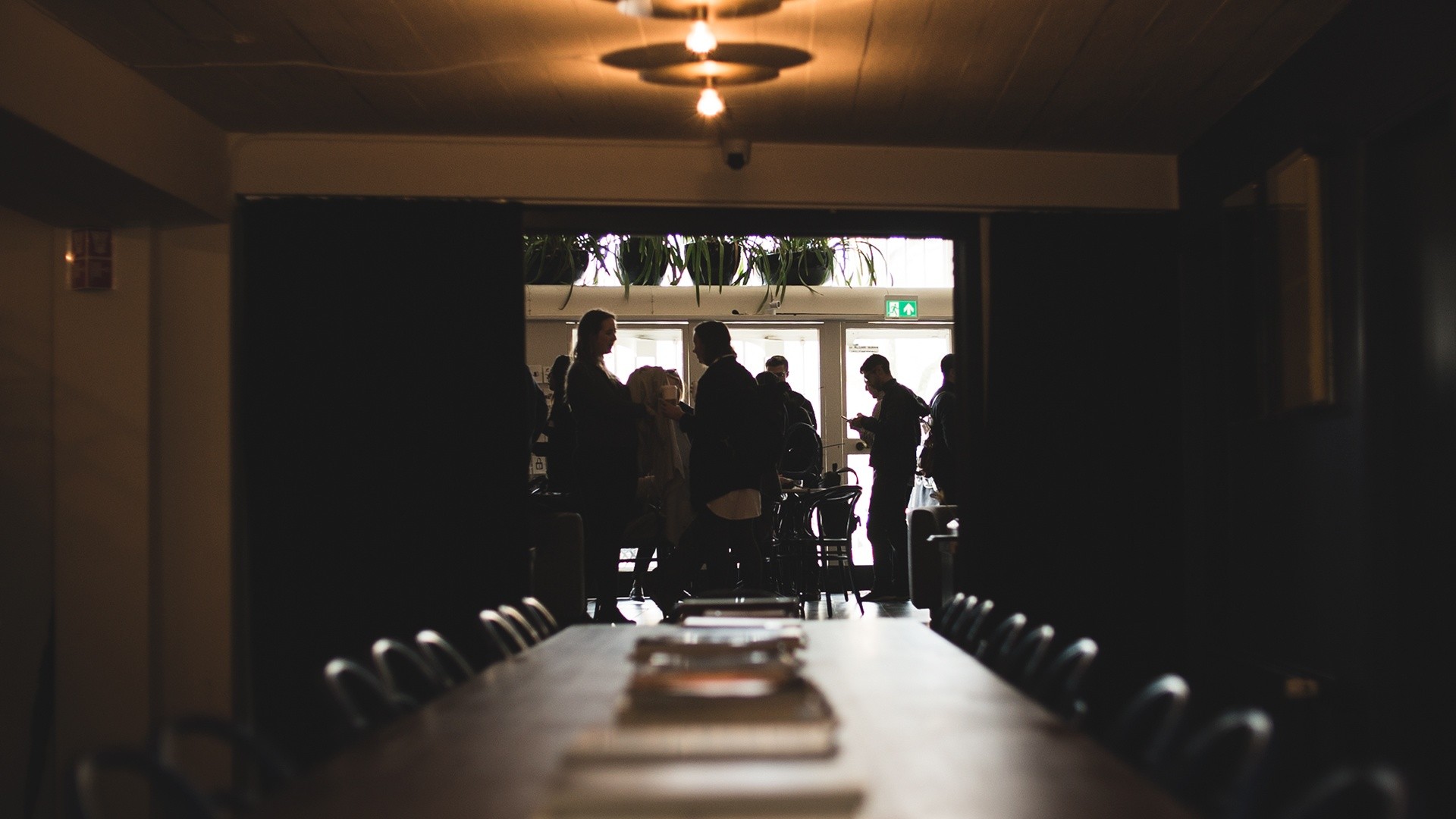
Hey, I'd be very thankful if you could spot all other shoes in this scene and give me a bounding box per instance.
[598,609,636,624]
[861,589,895,601]
[629,586,645,601]
[802,590,821,600]
[660,605,680,623]
[895,588,911,602]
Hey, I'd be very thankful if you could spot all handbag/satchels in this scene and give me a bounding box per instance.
[919,390,959,478]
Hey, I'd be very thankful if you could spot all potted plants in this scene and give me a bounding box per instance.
[522,233,888,313]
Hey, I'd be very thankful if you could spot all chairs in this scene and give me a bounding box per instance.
[72,597,560,819]
[774,486,1411,819]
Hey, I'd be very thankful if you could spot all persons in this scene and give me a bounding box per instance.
[756,371,822,602]
[524,366,547,493]
[766,356,817,430]
[629,370,694,600]
[563,311,652,625]
[926,354,971,505]
[658,321,782,597]
[532,356,573,492]
[849,355,921,602]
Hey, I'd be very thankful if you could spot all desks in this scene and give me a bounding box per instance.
[243,614,1202,819]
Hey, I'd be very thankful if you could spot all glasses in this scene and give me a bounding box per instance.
[775,372,787,378]
[864,368,877,383]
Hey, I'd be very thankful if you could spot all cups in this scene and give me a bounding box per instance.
[662,385,677,407]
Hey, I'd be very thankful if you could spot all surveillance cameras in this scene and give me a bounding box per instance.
[725,138,750,169]
